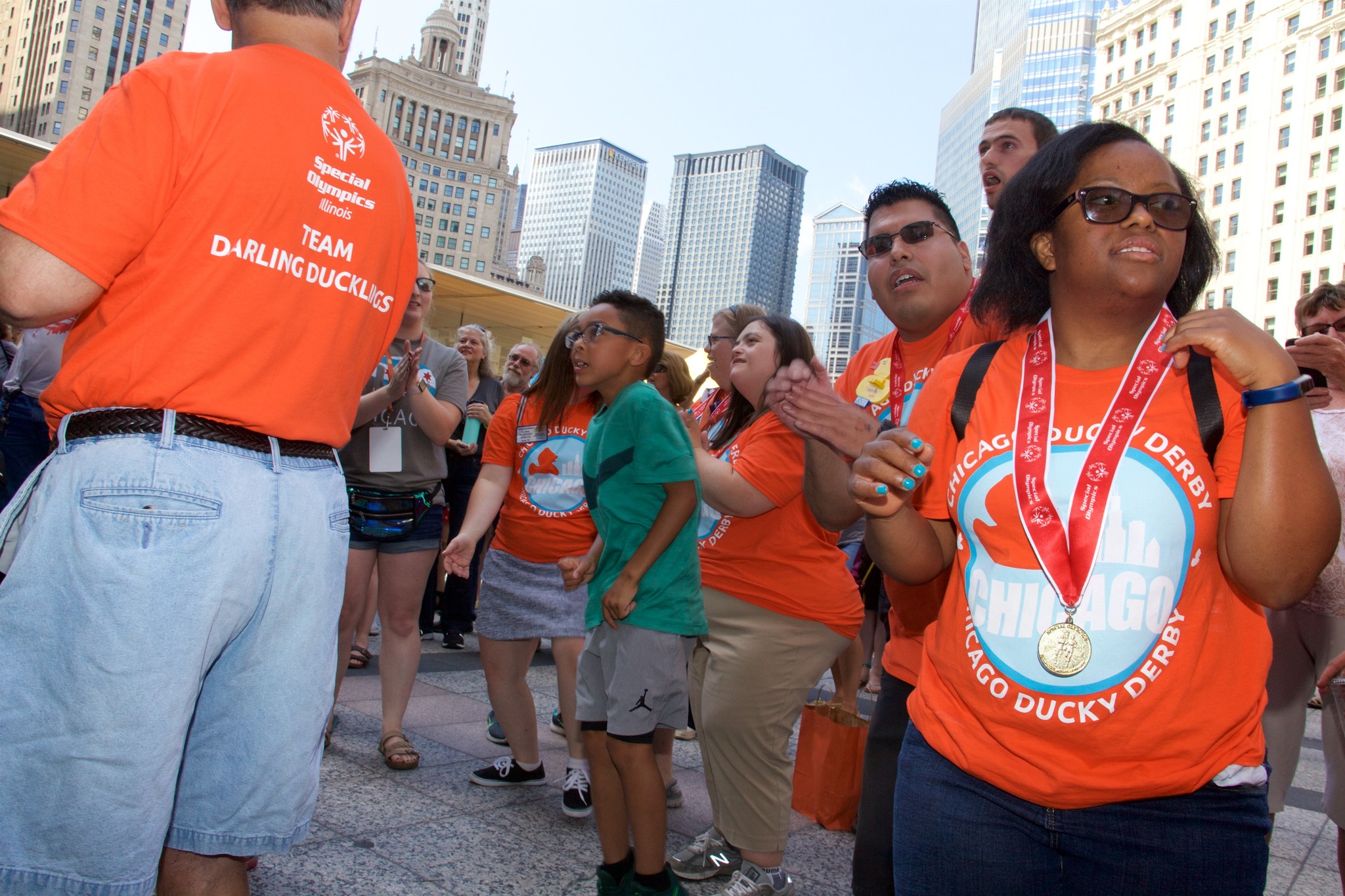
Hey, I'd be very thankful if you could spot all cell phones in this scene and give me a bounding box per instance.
[1285,338,1329,388]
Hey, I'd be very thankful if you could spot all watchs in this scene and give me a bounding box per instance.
[407,377,428,394]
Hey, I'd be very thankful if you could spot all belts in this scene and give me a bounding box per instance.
[47,410,339,466]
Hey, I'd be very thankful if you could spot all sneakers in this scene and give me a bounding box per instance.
[669,827,741,880]
[708,859,795,896]
[553,766,595,817]
[470,756,547,787]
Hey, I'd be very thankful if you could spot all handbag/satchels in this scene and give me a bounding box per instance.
[791,684,874,834]
[346,481,446,537]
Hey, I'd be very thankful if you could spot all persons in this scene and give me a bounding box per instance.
[678,317,868,896]
[0,314,79,515]
[568,287,706,896]
[435,304,769,824]
[836,117,1342,896]
[1256,281,1345,896]
[312,256,471,776]
[0,0,423,896]
[762,106,1061,895]
[344,555,381,669]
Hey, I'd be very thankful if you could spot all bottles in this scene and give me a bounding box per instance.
[461,398,485,445]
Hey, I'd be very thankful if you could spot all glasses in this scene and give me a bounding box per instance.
[707,335,736,349]
[477,325,486,333]
[416,277,436,292]
[1302,316,1345,336]
[509,354,533,367]
[1050,185,1199,231]
[654,364,666,373]
[858,220,955,259]
[565,323,643,348]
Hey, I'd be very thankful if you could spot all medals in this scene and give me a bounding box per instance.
[1036,616,1091,677]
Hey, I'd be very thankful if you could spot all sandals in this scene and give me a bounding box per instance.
[859,680,881,694]
[379,730,419,769]
[348,645,372,668]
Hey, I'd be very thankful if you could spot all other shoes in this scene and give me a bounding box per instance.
[667,780,685,809]
[418,628,466,650]
[596,848,689,896]
[551,707,565,736]
[675,727,698,740]
[487,711,507,742]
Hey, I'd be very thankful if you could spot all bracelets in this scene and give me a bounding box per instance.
[1241,374,1314,408]
